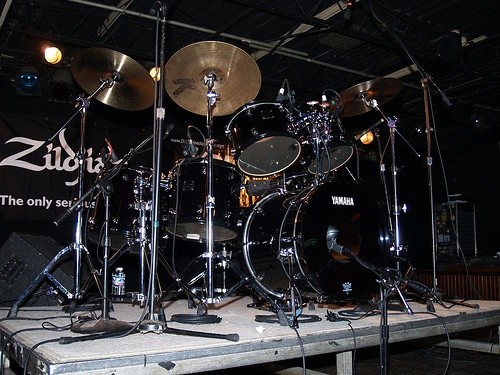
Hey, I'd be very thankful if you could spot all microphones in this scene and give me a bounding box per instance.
[276,86,284,100]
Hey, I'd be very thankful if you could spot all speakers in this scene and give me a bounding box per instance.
[0,231,88,308]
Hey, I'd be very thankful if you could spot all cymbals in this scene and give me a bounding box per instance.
[331,78,402,118]
[159,40,262,118]
[68,49,156,113]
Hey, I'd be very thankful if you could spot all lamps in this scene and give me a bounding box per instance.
[146,64,160,81]
[38,40,62,64]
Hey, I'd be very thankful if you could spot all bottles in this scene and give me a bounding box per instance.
[111,267,126,302]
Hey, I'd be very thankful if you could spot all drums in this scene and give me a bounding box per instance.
[84,163,165,257]
[226,100,303,181]
[241,176,416,305]
[161,155,243,242]
[306,113,355,175]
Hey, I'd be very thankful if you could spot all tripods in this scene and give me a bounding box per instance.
[0,84,440,345]
